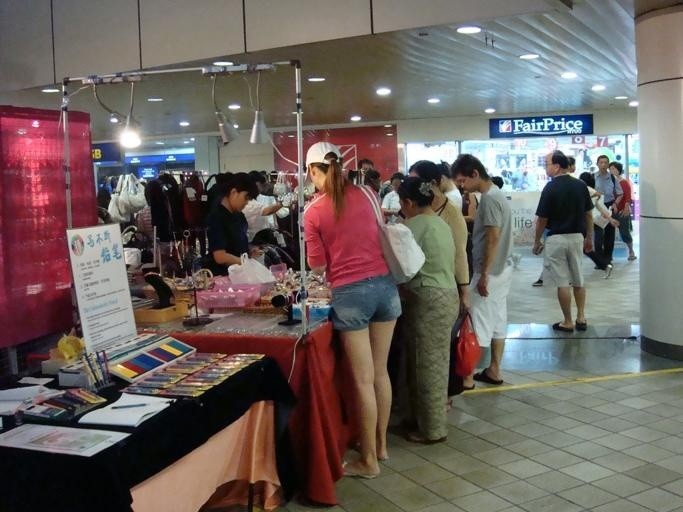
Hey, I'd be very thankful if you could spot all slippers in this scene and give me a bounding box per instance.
[340,461,378,479]
[354,441,390,462]
[553,322,574,332]
[576,319,587,329]
[473,367,505,384]
[456,377,474,389]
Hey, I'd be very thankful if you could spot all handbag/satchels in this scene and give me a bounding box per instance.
[272,170,288,195]
[118,174,138,215]
[455,309,482,377]
[106,175,131,222]
[128,173,148,208]
[357,182,427,283]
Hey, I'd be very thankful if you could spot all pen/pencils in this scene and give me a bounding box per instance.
[77,388,101,400]
[48,398,78,410]
[115,339,193,379]
[123,352,226,394]
[111,404,146,409]
[164,354,259,396]
[83,351,111,391]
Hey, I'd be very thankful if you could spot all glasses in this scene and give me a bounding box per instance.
[543,163,554,167]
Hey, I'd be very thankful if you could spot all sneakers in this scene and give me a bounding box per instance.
[603,263,613,279]
[532,280,544,287]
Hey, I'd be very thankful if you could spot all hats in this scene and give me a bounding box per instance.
[303,142,343,182]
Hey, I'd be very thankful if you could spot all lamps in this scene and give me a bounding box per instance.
[246,72,274,146]
[90,78,145,149]
[207,72,242,147]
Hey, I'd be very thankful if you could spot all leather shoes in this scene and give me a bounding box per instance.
[391,422,416,434]
[407,430,448,444]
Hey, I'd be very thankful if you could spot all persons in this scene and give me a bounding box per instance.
[532,173,620,287]
[591,155,623,269]
[206,177,258,277]
[396,177,460,444]
[610,162,636,260]
[451,154,511,390]
[532,150,593,331]
[567,158,575,176]
[242,170,283,242]
[364,168,380,191]
[357,160,374,185]
[437,163,462,215]
[409,161,471,396]
[305,142,402,478]
[381,173,404,223]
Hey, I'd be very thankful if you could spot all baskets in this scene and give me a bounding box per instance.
[193,284,260,307]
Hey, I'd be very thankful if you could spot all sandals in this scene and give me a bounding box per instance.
[627,255,637,261]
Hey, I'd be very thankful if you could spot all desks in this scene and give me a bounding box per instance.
[0,352,276,512]
[138,315,361,506]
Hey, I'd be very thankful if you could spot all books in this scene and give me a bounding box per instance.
[0,424,134,457]
[0,386,51,416]
[78,390,177,428]
